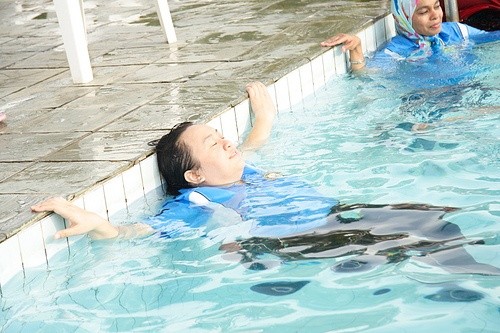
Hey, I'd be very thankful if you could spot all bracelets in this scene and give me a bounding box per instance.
[349,58,365,65]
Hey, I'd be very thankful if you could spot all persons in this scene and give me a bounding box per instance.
[320,0,500,132]
[30,81,500,303]
